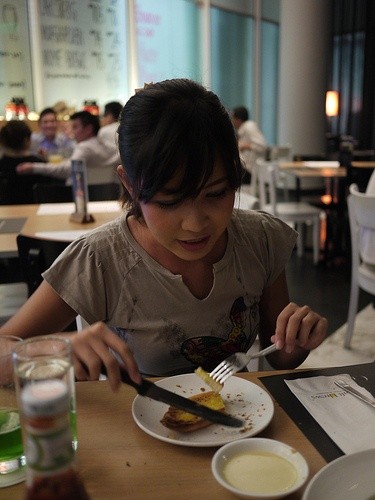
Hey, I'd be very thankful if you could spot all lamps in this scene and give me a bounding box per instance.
[326,91,341,116]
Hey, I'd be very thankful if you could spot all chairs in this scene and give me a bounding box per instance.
[344,184,375,350]
[15,234,77,333]
[270,144,292,162]
[256,157,320,264]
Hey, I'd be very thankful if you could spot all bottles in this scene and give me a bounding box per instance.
[22,380,91,499]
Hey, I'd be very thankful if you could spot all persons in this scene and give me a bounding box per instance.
[31,108,72,157]
[52,102,66,114]
[82,99,99,116]
[0,79,327,393]
[17,110,123,199]
[98,101,124,165]
[232,105,267,185]
[7,95,28,116]
[0,119,75,203]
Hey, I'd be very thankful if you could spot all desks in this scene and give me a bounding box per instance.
[275,160,375,264]
[0,200,132,285]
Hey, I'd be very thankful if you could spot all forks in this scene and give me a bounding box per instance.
[210,342,279,382]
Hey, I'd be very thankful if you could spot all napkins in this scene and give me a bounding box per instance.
[284,374,375,455]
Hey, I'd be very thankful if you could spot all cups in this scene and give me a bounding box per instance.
[13,336,78,450]
[0,335,23,487]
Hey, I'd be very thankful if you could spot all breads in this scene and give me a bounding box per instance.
[194,367,224,393]
[159,390,225,433]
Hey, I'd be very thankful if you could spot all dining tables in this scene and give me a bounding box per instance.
[0,363,374,500]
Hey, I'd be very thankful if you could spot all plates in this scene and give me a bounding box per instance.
[132,372,273,446]
[302,448,375,500]
[211,437,310,497]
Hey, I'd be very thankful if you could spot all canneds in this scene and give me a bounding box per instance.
[20,380,75,487]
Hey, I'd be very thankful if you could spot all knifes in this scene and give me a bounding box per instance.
[78,354,246,429]
